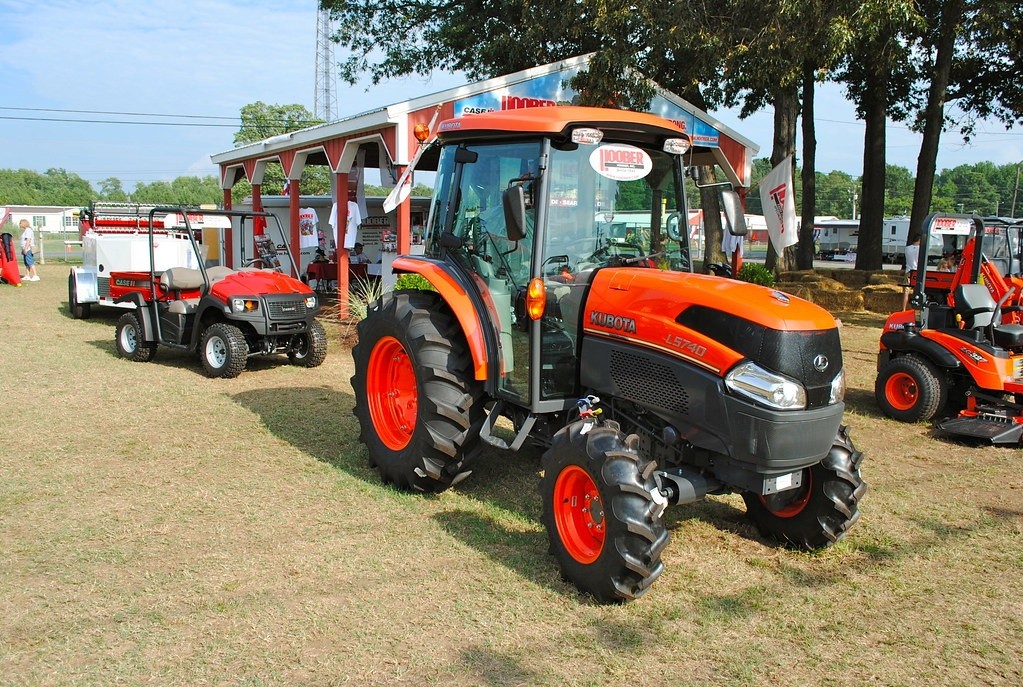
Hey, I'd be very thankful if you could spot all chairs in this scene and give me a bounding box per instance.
[953,284,1023,347]
[481,206,569,331]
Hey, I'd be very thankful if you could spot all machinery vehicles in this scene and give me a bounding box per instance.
[873,211,1023,447]
[350,105,867,606]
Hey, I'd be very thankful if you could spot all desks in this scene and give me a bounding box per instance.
[305,264,368,298]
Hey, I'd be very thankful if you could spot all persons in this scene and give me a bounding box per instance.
[479,162,604,395]
[937,257,957,273]
[19,219,40,281]
[903,234,922,311]
[354,243,372,263]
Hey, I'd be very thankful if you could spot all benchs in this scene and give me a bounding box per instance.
[160,265,280,314]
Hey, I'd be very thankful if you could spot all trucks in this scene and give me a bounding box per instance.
[68,202,203,319]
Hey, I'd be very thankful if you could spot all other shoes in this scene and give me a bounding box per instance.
[30,276,40,281]
[20,275,31,280]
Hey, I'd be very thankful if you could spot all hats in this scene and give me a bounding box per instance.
[353,243,364,249]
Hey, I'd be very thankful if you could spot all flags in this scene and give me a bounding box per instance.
[382,113,438,213]
[758,153,799,258]
